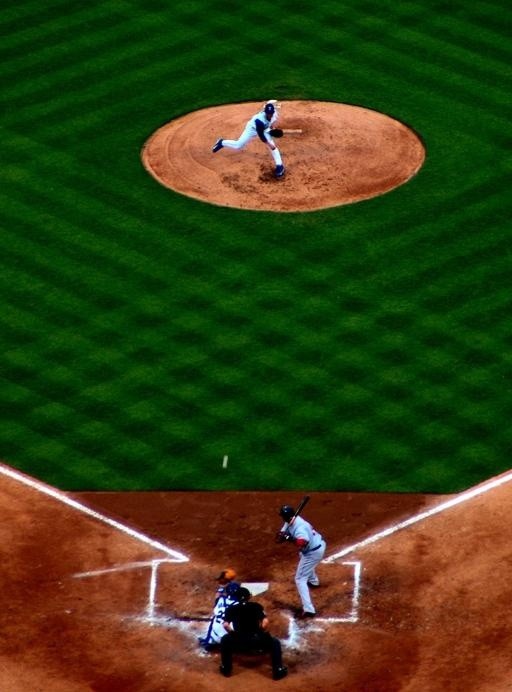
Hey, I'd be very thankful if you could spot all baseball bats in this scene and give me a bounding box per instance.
[278,495,308,539]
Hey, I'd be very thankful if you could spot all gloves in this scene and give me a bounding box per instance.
[275,531,298,544]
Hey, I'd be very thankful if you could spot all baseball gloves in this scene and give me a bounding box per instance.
[269,128,284,139]
[218,570,237,588]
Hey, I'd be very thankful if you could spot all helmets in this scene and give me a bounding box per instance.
[278,504,294,518]
[265,103,275,114]
[226,582,250,606]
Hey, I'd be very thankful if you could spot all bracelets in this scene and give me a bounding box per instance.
[216,587,225,593]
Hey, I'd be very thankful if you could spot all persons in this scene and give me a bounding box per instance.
[276,504,327,619]
[212,104,285,177]
[219,587,288,680]
[197,582,239,653]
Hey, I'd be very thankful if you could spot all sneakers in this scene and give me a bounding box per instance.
[275,165,285,177]
[219,664,233,676]
[272,665,288,678]
[294,609,317,619]
[212,138,224,152]
